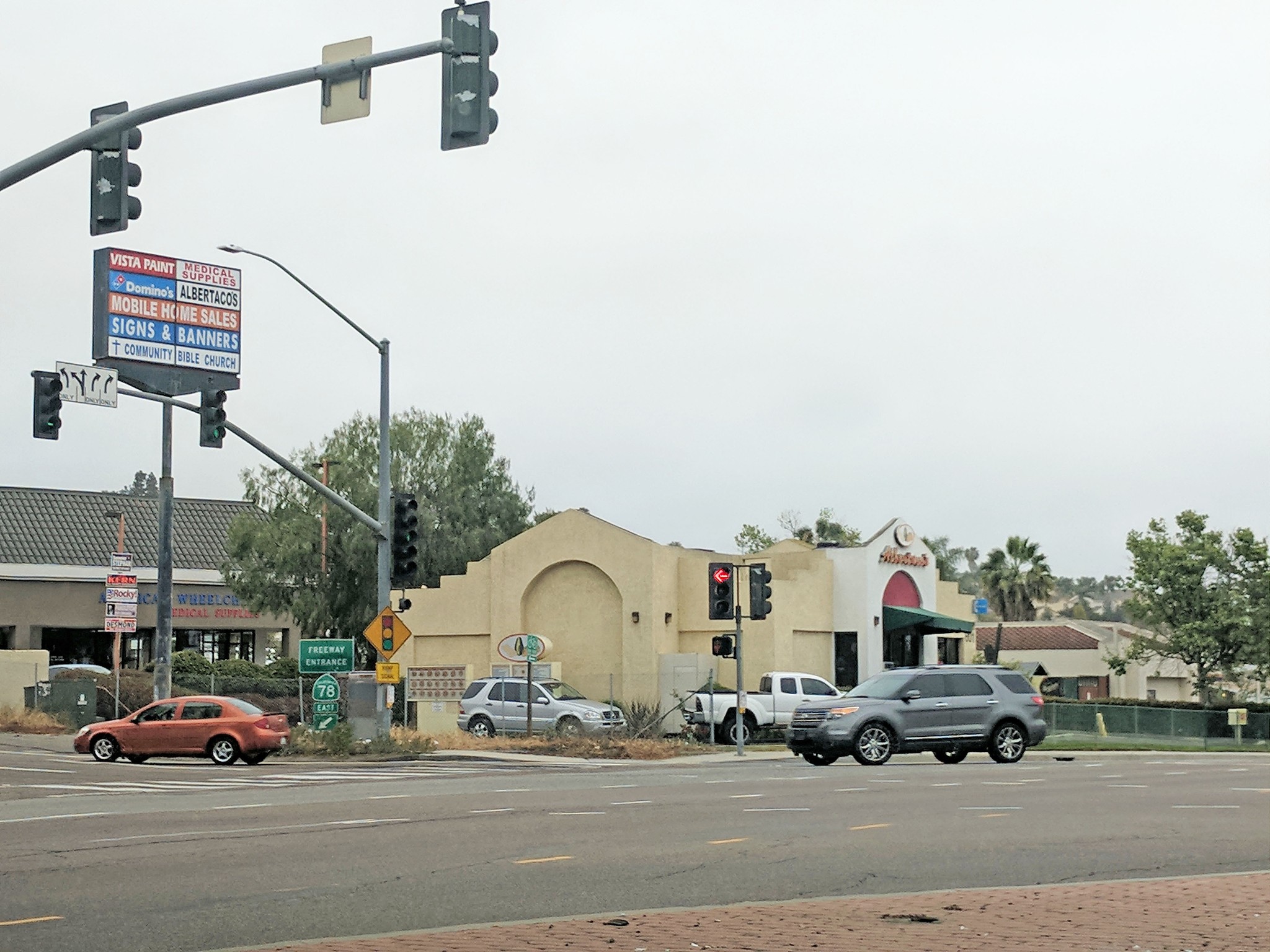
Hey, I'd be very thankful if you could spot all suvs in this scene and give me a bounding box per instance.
[457,677,628,742]
[785,664,1047,765]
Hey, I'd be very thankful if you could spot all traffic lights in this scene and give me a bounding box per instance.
[709,563,734,620]
[200,387,227,449]
[90,101,141,237]
[30,371,62,440]
[440,1,498,151]
[712,636,732,655]
[400,598,412,611]
[750,563,772,619]
[391,493,418,582]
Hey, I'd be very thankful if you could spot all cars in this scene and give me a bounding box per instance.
[75,695,290,765]
[49,663,111,682]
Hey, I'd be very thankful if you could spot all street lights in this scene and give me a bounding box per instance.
[217,243,392,665]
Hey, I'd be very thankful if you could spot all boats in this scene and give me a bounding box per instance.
[362,606,412,660]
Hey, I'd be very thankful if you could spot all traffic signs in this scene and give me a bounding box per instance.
[56,362,118,408]
[311,674,340,731]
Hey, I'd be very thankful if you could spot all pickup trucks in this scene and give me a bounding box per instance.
[682,671,847,746]
